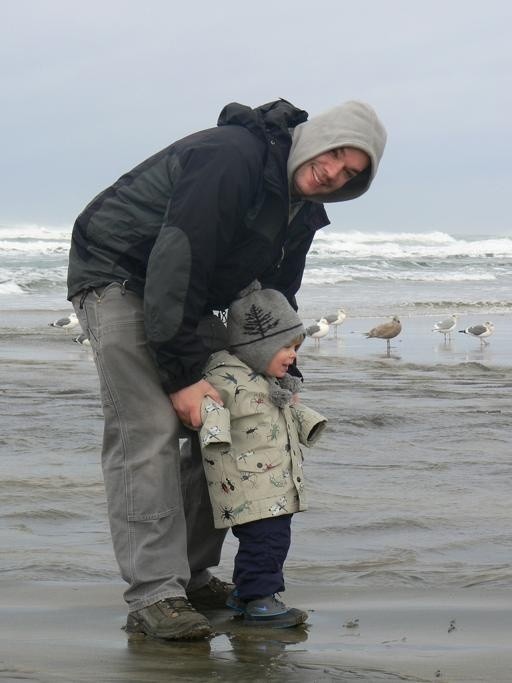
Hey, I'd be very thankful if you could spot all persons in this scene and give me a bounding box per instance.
[194,274,332,628]
[64,94,388,641]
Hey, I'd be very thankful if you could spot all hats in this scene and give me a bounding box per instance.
[227,280,307,407]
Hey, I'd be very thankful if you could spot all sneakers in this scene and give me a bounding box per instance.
[126,597,212,639]
[234,594,308,629]
[186,576,237,609]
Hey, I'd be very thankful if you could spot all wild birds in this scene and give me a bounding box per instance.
[71,334,89,346]
[458,320,494,345]
[362,316,402,357]
[432,314,457,341]
[316,308,346,339]
[305,318,329,346]
[47,313,79,335]
